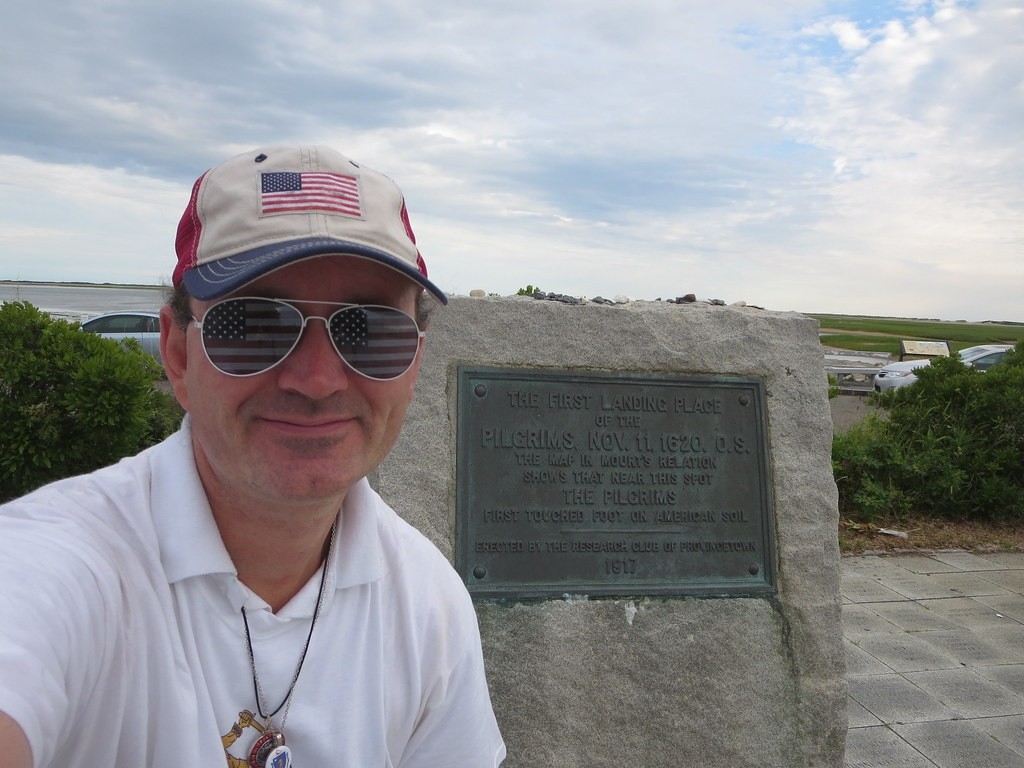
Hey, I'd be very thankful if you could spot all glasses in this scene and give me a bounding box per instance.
[185,296,428,381]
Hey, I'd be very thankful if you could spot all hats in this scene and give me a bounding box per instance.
[172,143,448,308]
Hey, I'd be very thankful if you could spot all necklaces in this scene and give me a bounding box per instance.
[240,523,335,768]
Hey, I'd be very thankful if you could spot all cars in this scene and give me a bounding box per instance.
[873,344,1016,399]
[77,311,169,378]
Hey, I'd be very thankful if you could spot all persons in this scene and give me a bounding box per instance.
[2,143,511,768]
